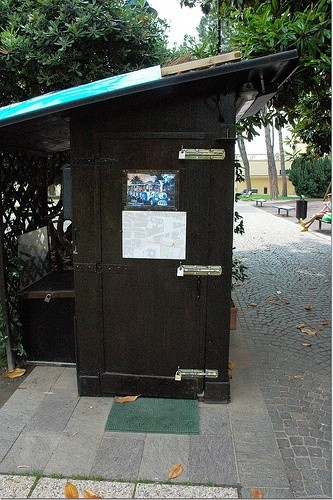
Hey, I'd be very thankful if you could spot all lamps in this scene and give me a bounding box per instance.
[236,82,259,124]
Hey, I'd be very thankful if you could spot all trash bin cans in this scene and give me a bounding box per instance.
[295,194,308,222]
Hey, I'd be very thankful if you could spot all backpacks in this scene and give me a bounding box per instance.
[322,212,332,223]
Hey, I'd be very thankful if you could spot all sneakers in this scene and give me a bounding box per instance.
[301,227,308,232]
[299,218,305,227]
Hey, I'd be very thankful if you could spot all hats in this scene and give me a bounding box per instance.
[327,193,331,196]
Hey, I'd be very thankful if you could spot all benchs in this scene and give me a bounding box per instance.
[310,216,331,230]
[272,205,294,217]
[255,200,267,207]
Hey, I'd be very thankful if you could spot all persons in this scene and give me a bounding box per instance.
[299,192,332,232]
